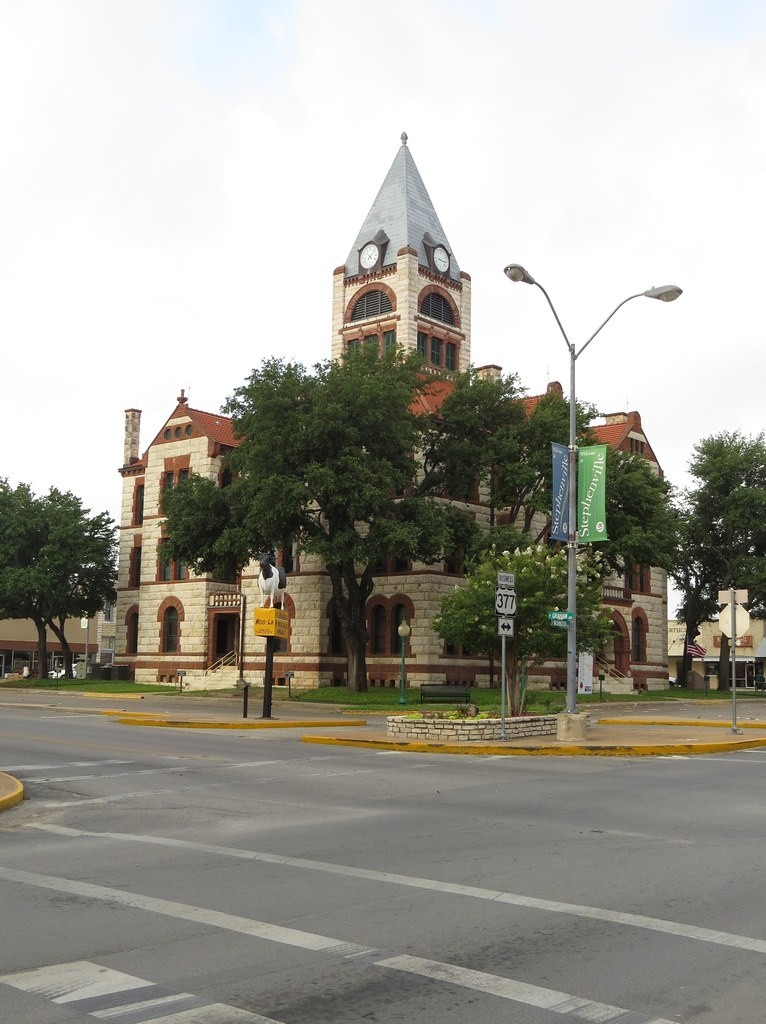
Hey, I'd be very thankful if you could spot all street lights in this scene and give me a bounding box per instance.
[505,263,684,722]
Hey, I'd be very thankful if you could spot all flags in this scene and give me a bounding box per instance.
[687,634,707,658]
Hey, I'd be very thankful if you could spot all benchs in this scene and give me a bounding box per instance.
[419,684,471,706]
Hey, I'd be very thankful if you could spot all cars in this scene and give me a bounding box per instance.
[669,676,676,686]
[48,664,77,679]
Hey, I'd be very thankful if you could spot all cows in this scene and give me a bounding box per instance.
[258,552,285,611]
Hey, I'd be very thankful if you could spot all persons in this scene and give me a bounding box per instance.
[22,664,31,678]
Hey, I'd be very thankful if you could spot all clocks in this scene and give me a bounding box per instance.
[433,247,450,273]
[360,244,379,270]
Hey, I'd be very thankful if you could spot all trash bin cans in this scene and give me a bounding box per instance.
[91,663,113,680]
[111,665,130,681]
